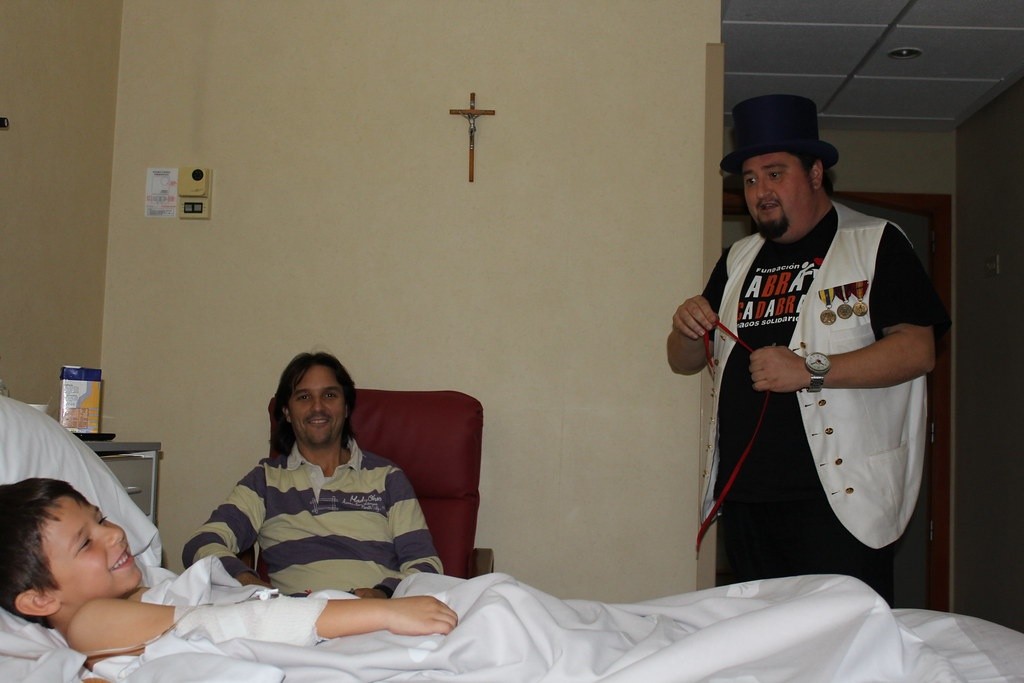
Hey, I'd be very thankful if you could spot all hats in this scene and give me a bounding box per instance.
[719,94,839,175]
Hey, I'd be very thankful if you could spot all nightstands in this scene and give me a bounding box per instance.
[79,438,162,529]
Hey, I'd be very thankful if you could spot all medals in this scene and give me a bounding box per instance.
[852,301,868,316]
[837,304,852,319]
[821,309,836,325]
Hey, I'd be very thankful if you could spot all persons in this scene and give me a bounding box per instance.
[665,95,951,610]
[181,353,443,599]
[0,477,459,668]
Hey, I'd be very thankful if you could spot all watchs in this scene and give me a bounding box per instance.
[804,352,831,393]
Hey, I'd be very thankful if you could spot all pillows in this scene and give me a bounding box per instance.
[0,392,165,661]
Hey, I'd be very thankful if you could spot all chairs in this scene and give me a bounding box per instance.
[235,387,497,580]
